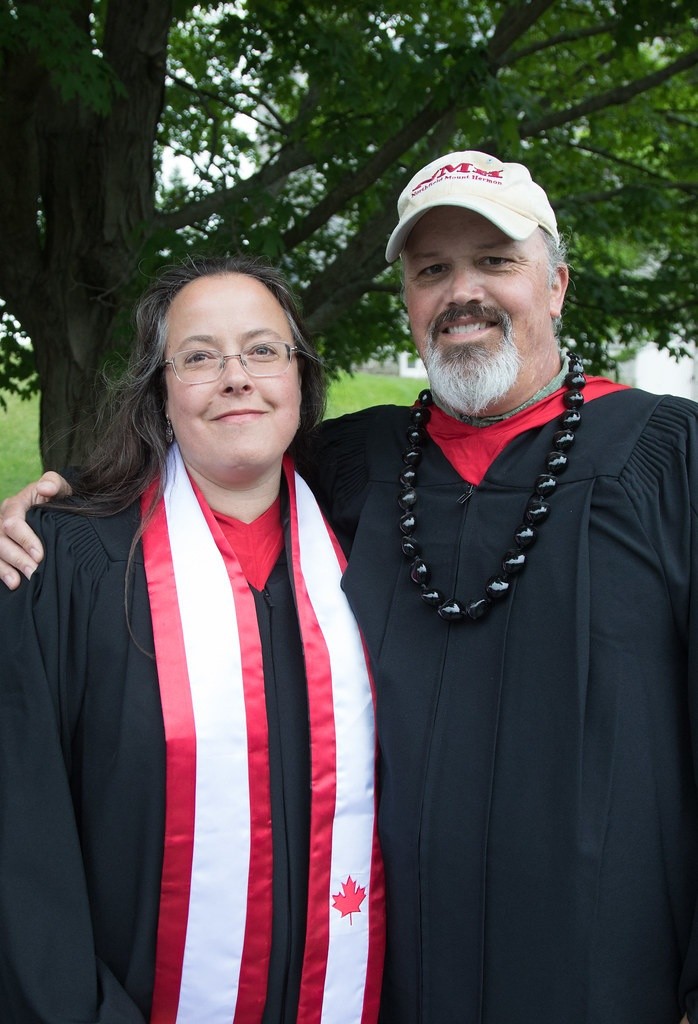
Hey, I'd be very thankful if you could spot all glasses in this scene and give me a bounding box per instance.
[155,340,301,385]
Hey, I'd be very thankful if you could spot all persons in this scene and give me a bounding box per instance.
[0,248,391,1022]
[0,148,696,1024]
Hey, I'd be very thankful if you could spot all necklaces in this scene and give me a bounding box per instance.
[395,343,585,629]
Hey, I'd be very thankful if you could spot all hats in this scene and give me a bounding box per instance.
[385,149,559,264]
